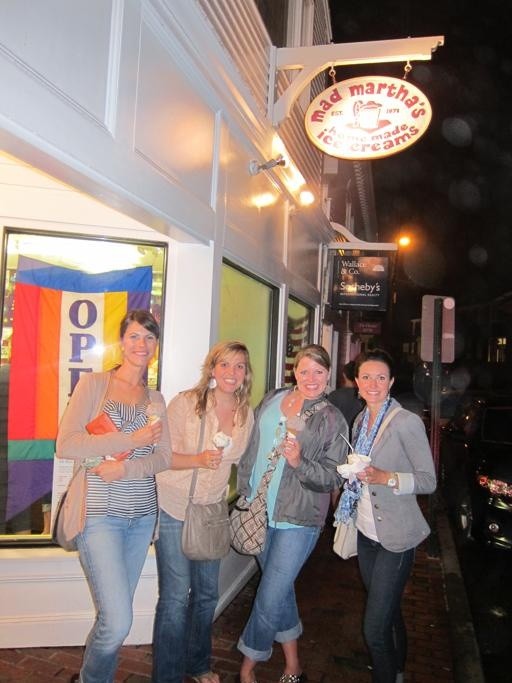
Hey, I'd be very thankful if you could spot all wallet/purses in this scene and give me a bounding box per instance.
[86,412,130,460]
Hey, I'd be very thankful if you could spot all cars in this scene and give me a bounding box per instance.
[412,359,491,416]
[439,373,510,555]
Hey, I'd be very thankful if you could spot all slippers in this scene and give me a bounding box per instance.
[276,670,309,682]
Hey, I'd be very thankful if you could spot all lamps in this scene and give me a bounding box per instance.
[247,150,289,177]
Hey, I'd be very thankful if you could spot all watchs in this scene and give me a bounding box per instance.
[389,472,399,491]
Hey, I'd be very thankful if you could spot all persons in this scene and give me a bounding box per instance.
[53,309,172,683]
[233,344,350,682]
[337,353,441,681]
[152,339,254,683]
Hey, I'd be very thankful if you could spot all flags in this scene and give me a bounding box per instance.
[3,255,154,521]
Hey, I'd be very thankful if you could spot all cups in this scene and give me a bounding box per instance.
[358,103,381,126]
[347,453,371,467]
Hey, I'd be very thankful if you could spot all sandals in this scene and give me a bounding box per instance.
[192,670,221,683]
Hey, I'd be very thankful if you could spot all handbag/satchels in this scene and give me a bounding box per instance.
[51,491,80,552]
[230,494,269,554]
[180,499,231,561]
[332,514,359,560]
